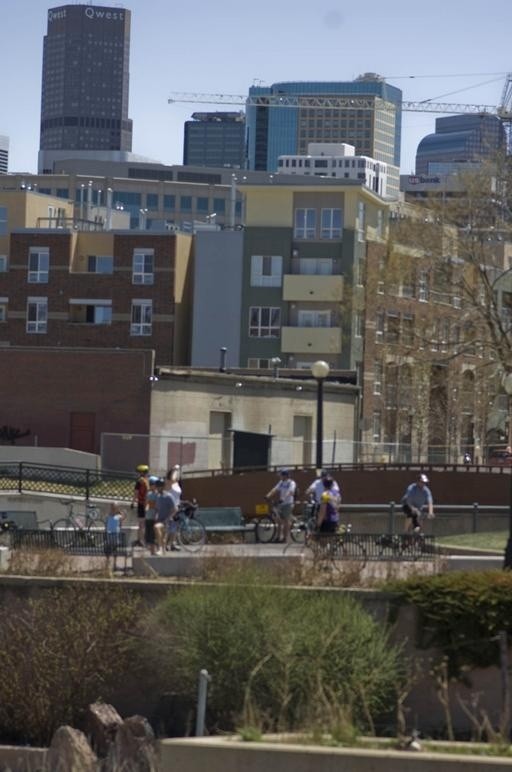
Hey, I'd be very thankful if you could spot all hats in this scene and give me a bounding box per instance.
[278,469,288,475]
[418,472,430,483]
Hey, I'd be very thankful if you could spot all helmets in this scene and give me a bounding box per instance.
[322,477,335,488]
[135,464,165,487]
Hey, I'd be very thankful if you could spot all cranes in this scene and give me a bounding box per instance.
[163,68,512,125]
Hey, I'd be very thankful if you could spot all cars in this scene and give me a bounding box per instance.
[487,449,512,465]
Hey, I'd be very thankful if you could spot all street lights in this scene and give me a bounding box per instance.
[308,359,330,467]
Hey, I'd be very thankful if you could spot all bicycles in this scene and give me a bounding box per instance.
[47,495,108,548]
[180,499,208,544]
[175,507,208,553]
[254,498,312,548]
[303,495,321,549]
[390,513,429,562]
[282,522,367,576]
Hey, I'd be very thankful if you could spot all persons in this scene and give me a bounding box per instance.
[400,474,436,534]
[305,470,340,518]
[100,502,127,571]
[130,463,183,556]
[266,470,297,543]
[315,477,342,548]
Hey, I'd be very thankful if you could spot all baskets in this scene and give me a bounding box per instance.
[256,503,269,515]
